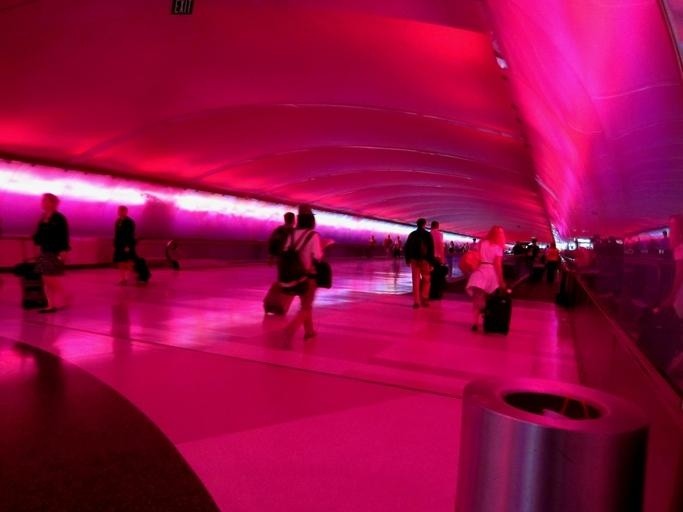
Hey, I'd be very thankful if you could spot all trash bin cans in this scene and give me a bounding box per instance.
[460,376,652,510]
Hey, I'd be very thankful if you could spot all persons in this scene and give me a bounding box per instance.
[463,226,507,330]
[112,206,135,284]
[267,213,295,267]
[283,203,324,344]
[32,194,70,313]
[368,233,401,258]
[511,239,560,285]
[403,218,434,307]
[449,238,476,253]
[428,221,444,265]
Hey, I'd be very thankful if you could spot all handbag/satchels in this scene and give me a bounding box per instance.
[32,251,66,276]
[458,248,480,275]
[313,261,332,289]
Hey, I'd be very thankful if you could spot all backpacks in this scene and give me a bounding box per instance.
[268,226,289,258]
[278,228,317,295]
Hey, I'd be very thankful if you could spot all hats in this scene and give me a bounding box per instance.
[296,205,316,215]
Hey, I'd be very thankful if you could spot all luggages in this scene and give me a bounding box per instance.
[482,293,512,334]
[262,281,295,315]
[132,256,150,282]
